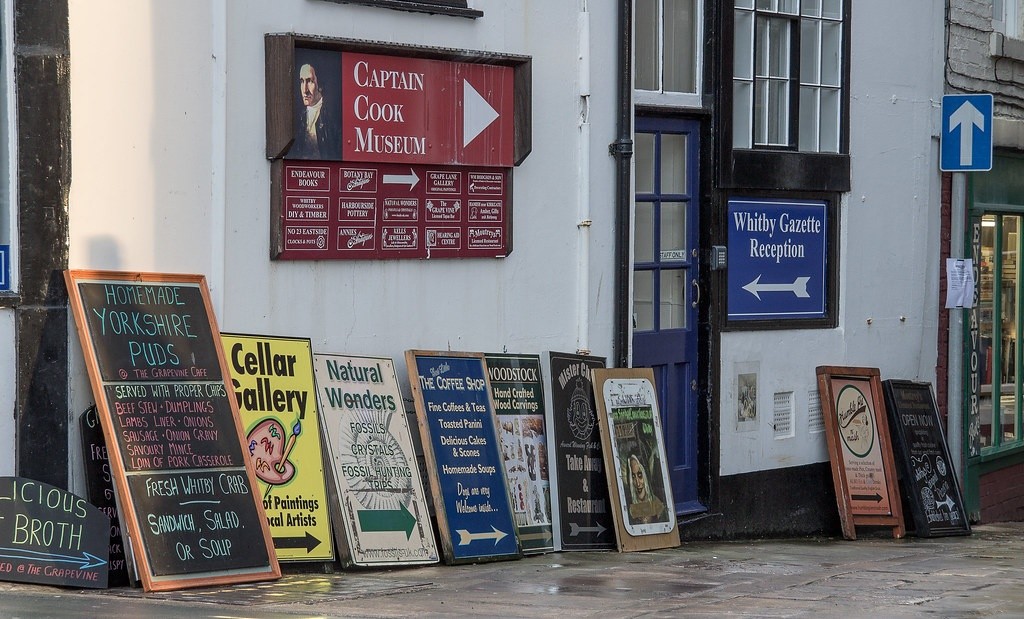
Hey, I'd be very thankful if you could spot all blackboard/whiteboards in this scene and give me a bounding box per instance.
[0,476,113,591]
[880,378,973,539]
[61,268,283,594]
